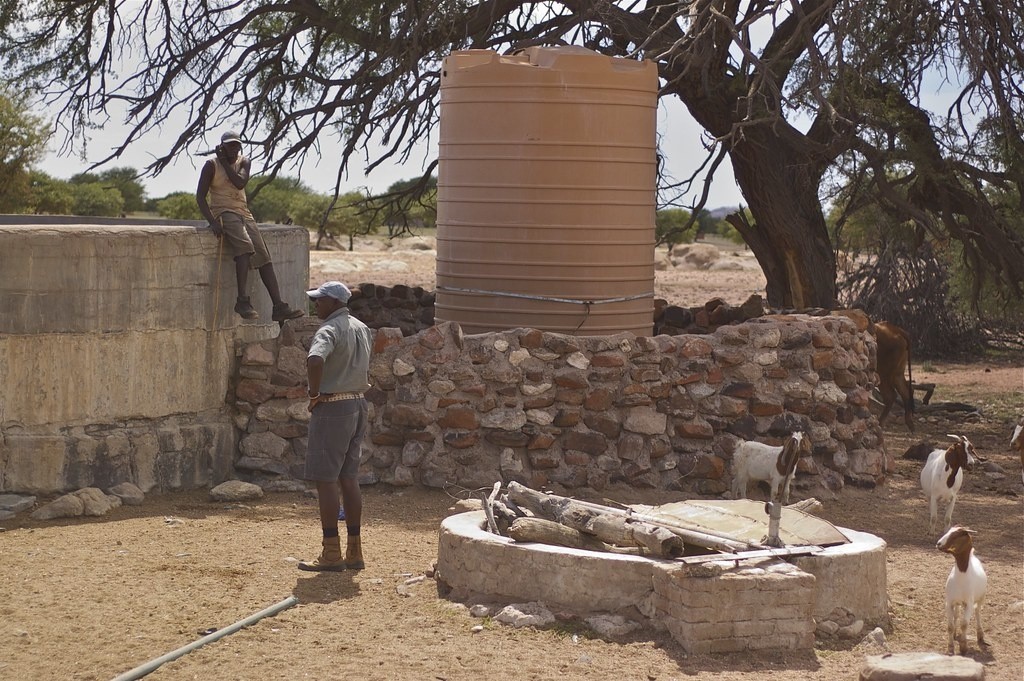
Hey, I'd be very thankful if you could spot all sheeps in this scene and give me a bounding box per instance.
[1007,423,1024,484]
[935,523,987,657]
[920,433,978,543]
[730,430,814,507]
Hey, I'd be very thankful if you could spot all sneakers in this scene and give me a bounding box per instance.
[272,302,305,321]
[234,296,259,319]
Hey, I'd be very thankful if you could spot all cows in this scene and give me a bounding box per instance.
[874,319,915,432]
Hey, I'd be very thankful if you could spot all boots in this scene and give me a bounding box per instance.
[343,535,365,568]
[298,536,345,571]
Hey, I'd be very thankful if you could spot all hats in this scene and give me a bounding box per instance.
[306,281,352,304]
[221,130,242,144]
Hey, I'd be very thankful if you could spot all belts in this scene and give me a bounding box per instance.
[319,391,364,402]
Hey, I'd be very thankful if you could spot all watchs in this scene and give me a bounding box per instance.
[307,390,320,399]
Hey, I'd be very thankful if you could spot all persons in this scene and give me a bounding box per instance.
[299,281,372,571]
[197,131,305,321]
